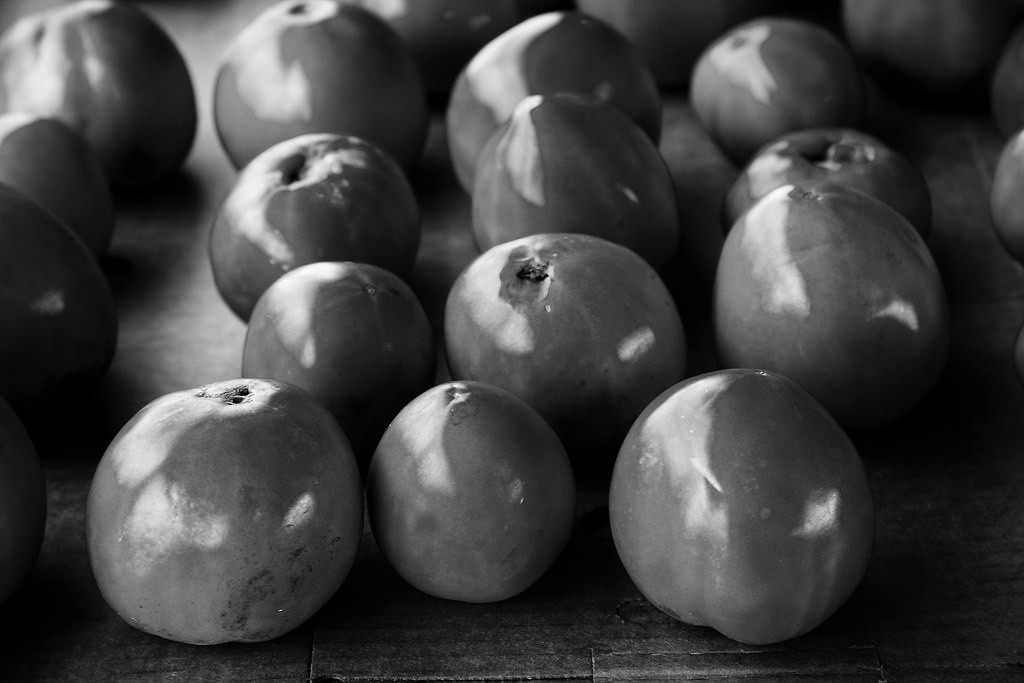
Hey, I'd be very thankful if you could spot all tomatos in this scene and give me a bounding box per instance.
[0,0,1024,647]
